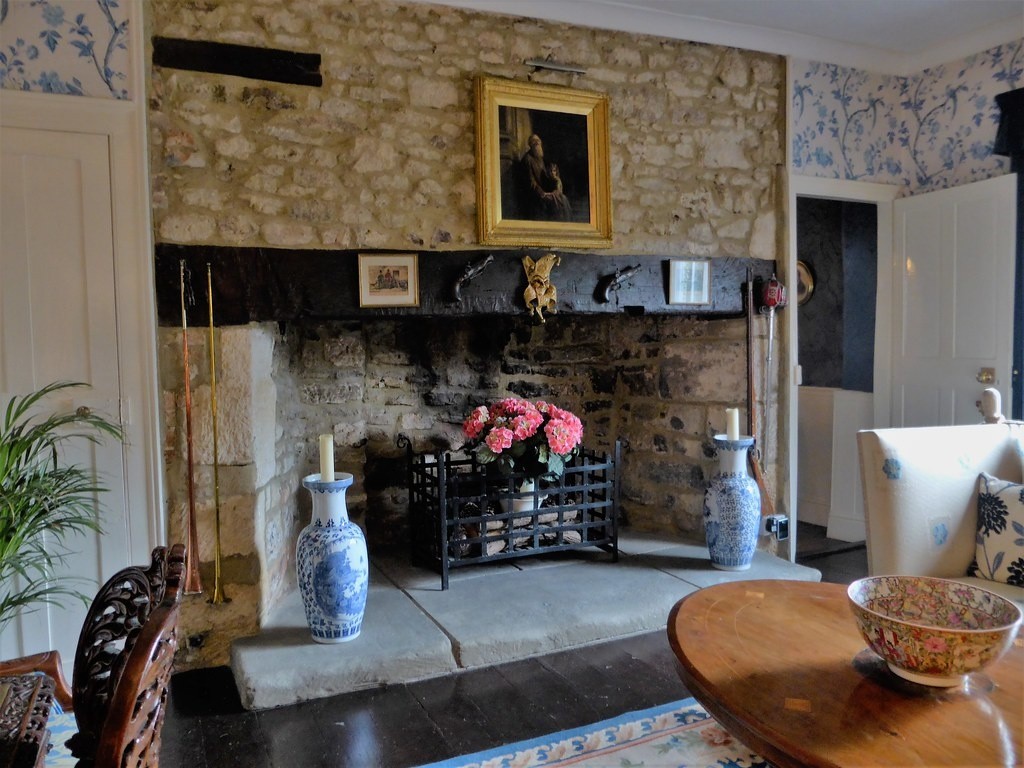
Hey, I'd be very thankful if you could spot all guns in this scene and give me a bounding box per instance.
[601,264,643,302]
[451,254,494,301]
[745,265,776,516]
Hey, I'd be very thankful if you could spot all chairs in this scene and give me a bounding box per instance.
[0,543,188,768]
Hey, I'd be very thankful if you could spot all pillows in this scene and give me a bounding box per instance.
[966,472,1024,589]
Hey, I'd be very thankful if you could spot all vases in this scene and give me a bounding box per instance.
[295,472,369,643]
[701,434,765,569]
[496,475,550,518]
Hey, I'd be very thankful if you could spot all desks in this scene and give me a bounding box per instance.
[668,578,1023,768]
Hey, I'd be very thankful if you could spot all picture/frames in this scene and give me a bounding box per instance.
[357,253,421,309]
[797,259,815,307]
[473,73,615,251]
[667,257,713,306]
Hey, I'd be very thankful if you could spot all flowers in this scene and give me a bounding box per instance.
[463,397,584,481]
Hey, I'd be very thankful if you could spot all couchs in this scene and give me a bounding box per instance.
[854,388,1024,626]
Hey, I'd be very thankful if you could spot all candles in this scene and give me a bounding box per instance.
[319,433,336,483]
[726,407,740,442]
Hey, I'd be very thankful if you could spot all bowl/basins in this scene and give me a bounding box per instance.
[845,574,1021,688]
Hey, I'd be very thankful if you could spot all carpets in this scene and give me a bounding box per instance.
[407,696,781,767]
